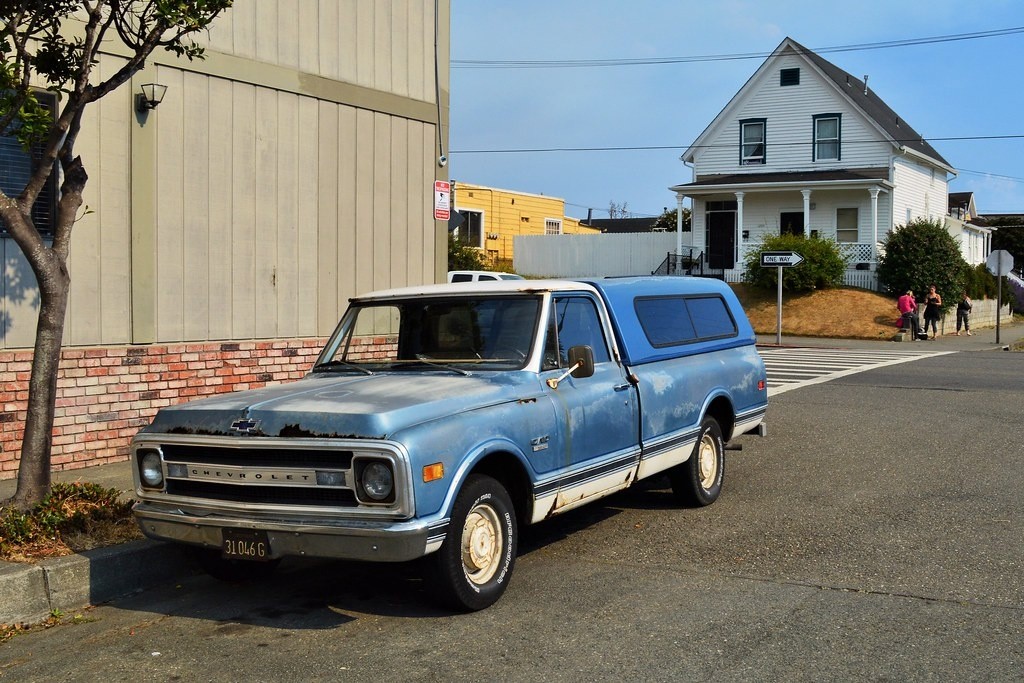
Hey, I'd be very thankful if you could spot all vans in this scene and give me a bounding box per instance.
[447,271,524,336]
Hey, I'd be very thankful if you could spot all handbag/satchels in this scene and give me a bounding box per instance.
[962,296,971,311]
[895,316,903,328]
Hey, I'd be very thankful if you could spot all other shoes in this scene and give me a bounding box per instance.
[957,333,961,336]
[915,338,921,341]
[967,333,972,336]
[931,337,937,340]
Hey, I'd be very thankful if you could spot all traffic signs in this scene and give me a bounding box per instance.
[759,251,805,267]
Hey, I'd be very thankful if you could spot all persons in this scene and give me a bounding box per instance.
[923,285,942,340]
[957,291,973,336]
[896,290,925,342]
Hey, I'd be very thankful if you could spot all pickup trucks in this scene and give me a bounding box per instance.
[130,275,768,612]
